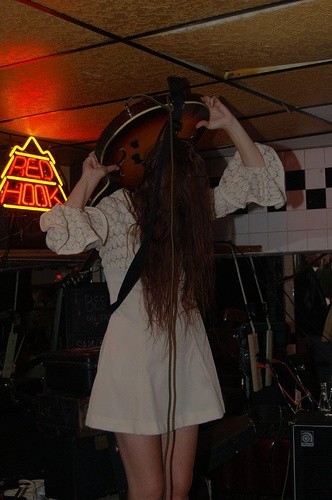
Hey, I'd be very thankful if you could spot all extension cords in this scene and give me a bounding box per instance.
[229,307,293,401]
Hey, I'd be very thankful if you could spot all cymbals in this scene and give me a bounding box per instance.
[57,343,102,356]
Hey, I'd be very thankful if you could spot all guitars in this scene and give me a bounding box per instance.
[51,89,211,291]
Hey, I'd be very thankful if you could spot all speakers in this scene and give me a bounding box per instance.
[292,413,332,500]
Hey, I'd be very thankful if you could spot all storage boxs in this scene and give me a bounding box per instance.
[44,345,101,396]
[37,390,91,429]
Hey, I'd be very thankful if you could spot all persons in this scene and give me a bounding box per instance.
[39,95,287,499]
